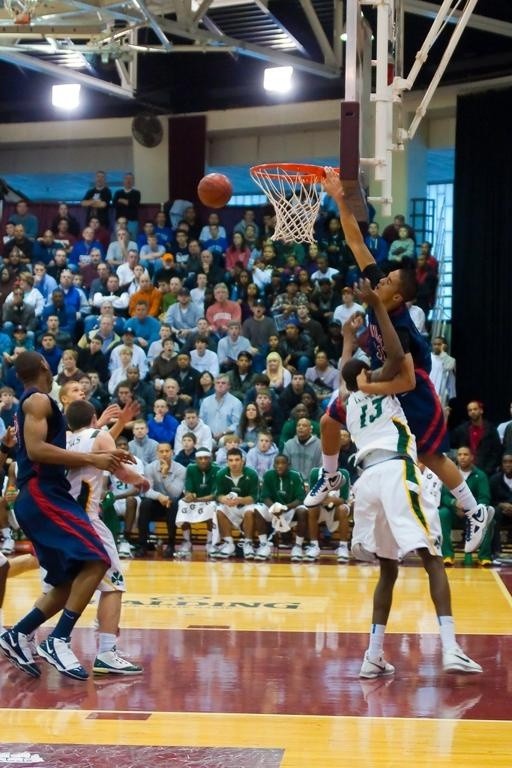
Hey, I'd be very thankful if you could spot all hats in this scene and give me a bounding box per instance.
[286,314,299,326]
[178,286,191,297]
[13,324,27,334]
[120,326,136,337]
[341,286,353,293]
[297,293,309,306]
[162,252,174,262]
[182,431,197,444]
[252,297,267,307]
[286,275,301,286]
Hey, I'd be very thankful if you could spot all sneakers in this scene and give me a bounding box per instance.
[253,541,275,562]
[359,650,395,679]
[134,542,148,559]
[300,466,347,508]
[0,625,42,680]
[460,502,497,555]
[238,537,255,561]
[442,644,484,674]
[160,542,176,558]
[303,541,321,563]
[334,543,351,563]
[92,643,144,676]
[215,540,237,559]
[476,555,503,568]
[0,535,15,557]
[442,552,455,566]
[289,543,304,563]
[117,537,134,560]
[35,633,90,681]
[172,538,194,560]
[205,539,221,558]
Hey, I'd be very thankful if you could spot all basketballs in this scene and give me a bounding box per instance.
[198,172,232,212]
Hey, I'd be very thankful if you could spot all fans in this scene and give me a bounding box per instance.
[132,112,163,147]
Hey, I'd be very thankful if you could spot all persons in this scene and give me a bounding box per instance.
[97,435,145,558]
[0,351,138,682]
[438,445,498,566]
[496,403,512,451]
[415,253,434,309]
[138,351,360,563]
[427,336,458,409]
[416,457,442,509]
[487,451,512,566]
[338,276,484,678]
[303,167,497,555]
[7,346,29,400]
[303,274,374,360]
[62,399,150,676]
[417,242,440,276]
[0,387,20,429]
[46,351,139,458]
[303,207,417,275]
[3,460,28,540]
[447,400,502,474]
[1,415,18,637]
[1,172,304,352]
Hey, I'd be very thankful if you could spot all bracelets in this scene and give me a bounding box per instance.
[1,444,17,455]
